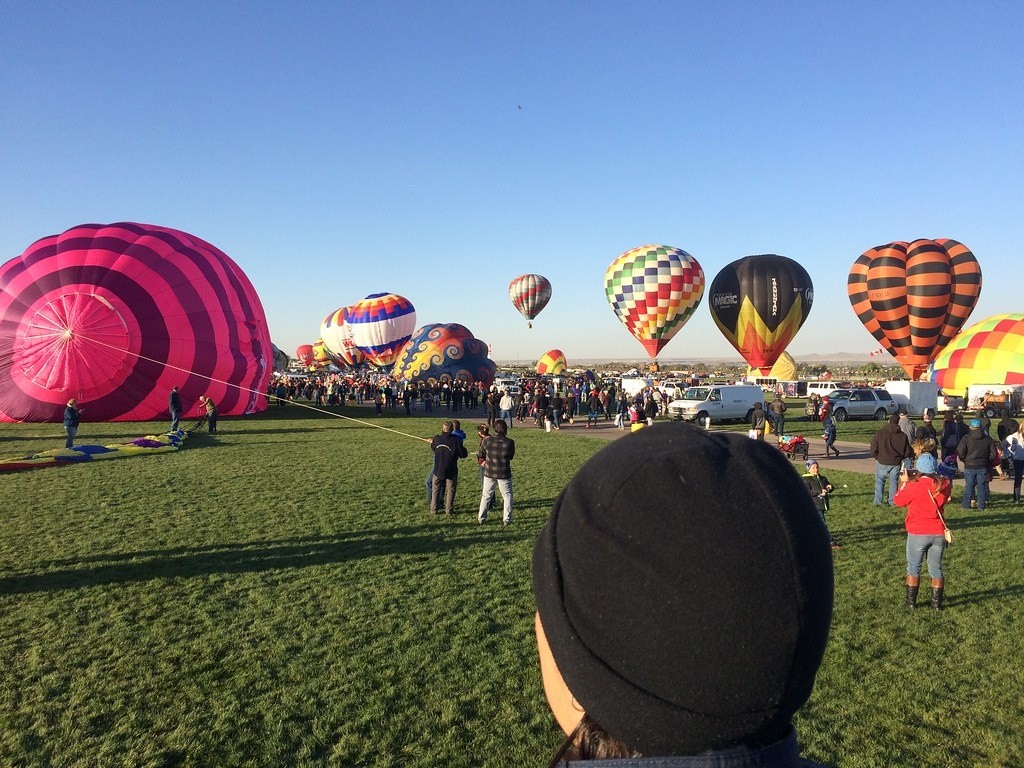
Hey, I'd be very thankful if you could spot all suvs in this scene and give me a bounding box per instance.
[806,387,896,422]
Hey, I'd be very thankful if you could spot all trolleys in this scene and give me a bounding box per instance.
[777,442,809,460]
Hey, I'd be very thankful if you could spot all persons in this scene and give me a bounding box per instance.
[426,419,467,510]
[427,420,468,515]
[476,420,516,526]
[477,423,497,510]
[266,367,668,433]
[64,399,85,448]
[532,420,834,768]
[753,386,1024,549]
[199,396,218,433]
[169,386,182,431]
[894,453,952,611]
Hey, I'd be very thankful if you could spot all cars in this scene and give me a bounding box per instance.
[494,379,522,395]
[654,380,688,396]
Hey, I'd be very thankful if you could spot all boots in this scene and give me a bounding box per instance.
[926,576,944,610]
[903,575,920,610]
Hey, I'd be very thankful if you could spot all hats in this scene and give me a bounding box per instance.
[916,452,937,473]
[531,426,835,756]
[890,415,900,423]
[970,419,981,427]
[805,459,819,471]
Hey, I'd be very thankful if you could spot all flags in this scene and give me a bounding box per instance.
[869,348,883,358]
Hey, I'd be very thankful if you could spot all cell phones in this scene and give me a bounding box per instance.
[906,469,917,476]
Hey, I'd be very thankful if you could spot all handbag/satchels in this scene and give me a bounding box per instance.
[749,429,758,440]
[944,528,954,544]
[824,435,828,440]
[944,435,958,448]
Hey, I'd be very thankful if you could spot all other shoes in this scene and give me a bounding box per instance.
[823,453,829,457]
[836,450,839,456]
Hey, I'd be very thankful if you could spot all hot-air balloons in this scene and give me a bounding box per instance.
[918,313,1024,398]
[848,237,983,382]
[708,254,814,377]
[746,351,798,381]
[509,273,552,329]
[0,222,273,422]
[271,292,495,391]
[604,243,705,372]
[536,348,571,377]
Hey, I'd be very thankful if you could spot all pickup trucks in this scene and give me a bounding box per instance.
[936,395,964,412]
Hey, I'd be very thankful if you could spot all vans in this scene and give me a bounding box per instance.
[806,381,844,397]
[666,385,764,426]
